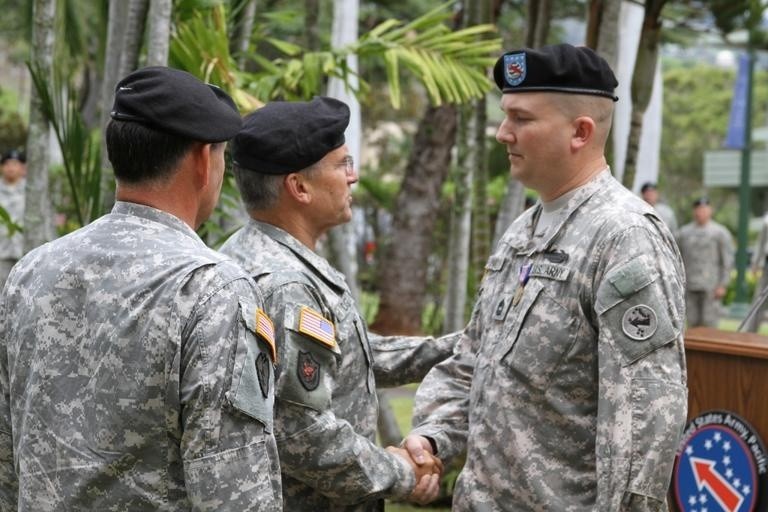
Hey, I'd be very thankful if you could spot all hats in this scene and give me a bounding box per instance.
[494,44,618,101]
[235,98,350,172]
[110,66,243,142]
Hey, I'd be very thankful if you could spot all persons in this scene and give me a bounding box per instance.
[638,178,679,237]
[221,96,438,512]
[0,146,31,281]
[670,196,737,329]
[1,65,288,512]
[752,205,767,322]
[358,221,376,278]
[408,43,690,512]
